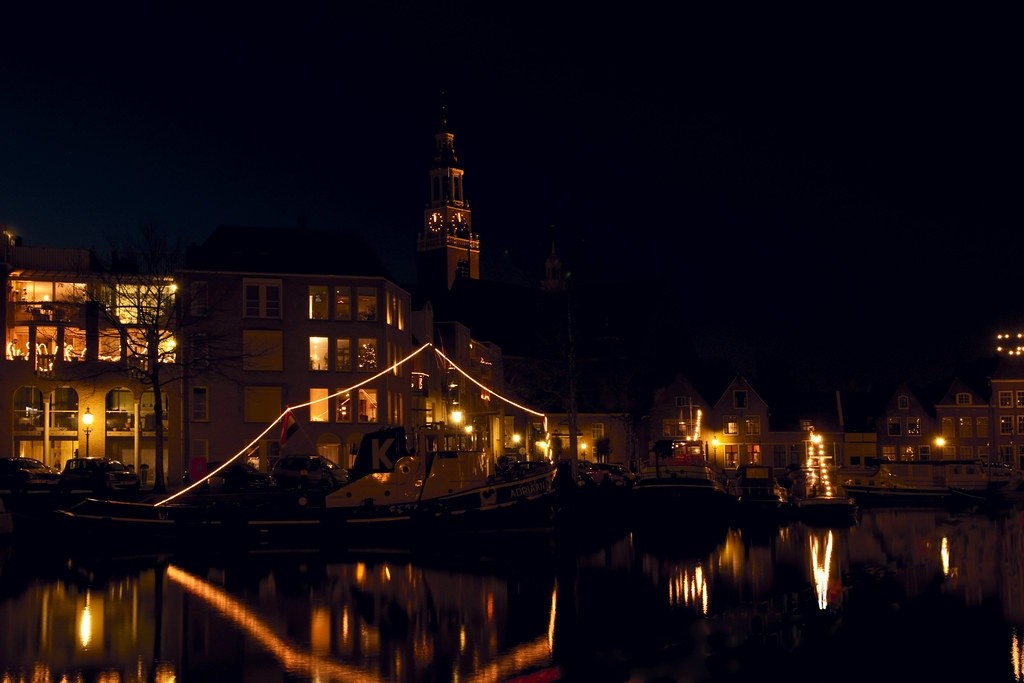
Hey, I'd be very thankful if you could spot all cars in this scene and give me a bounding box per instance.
[182,460,278,496]
[0,454,63,495]
[554,458,604,485]
[272,455,349,489]
[597,463,634,483]
[59,457,140,495]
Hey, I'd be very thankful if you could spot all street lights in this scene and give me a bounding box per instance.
[82,406,94,457]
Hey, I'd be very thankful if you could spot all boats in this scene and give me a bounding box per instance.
[840,459,1010,509]
[59,435,555,550]
[629,440,730,524]
[726,465,788,533]
